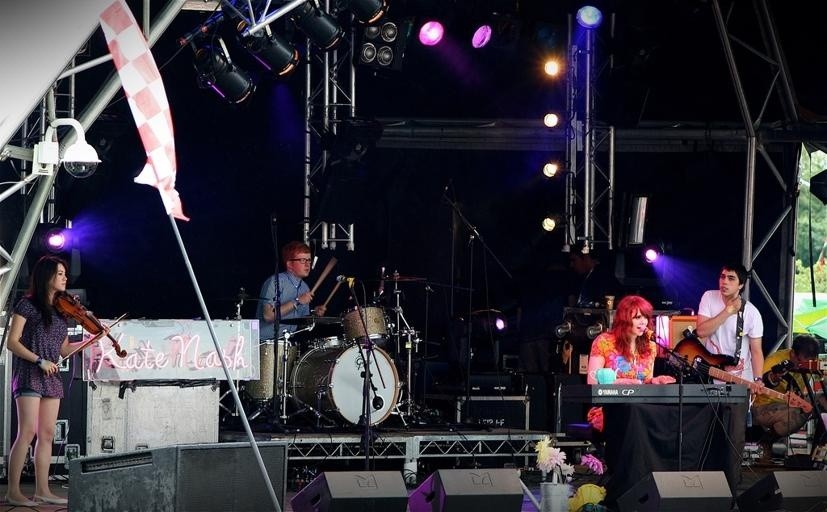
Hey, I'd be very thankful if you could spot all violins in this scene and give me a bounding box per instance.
[54,290,126,358]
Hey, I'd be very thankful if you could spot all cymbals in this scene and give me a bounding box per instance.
[279,316,345,324]
[364,276,427,282]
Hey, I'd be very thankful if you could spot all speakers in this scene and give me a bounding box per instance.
[617,470,733,512]
[67,440,288,512]
[408,469,524,512]
[289,471,409,512]
[736,471,827,512]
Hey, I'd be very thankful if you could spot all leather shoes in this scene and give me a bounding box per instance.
[33,494,69,503]
[4,495,39,507]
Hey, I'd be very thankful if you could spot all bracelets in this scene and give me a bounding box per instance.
[753,377,762,381]
[294,297,299,304]
[34,356,43,367]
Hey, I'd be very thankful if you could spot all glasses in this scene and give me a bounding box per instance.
[288,257,311,263]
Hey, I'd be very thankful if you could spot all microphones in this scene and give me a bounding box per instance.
[640,325,661,339]
[336,275,360,283]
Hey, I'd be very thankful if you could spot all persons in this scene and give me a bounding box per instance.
[752,336,827,460]
[696,262,765,485]
[5,256,111,507]
[569,243,621,310]
[586,294,677,384]
[255,240,326,343]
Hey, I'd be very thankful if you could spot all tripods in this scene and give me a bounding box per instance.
[443,235,493,432]
[390,404,410,431]
[394,334,441,424]
[247,338,335,425]
[219,305,242,417]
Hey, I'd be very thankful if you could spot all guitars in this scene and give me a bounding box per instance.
[672,335,814,413]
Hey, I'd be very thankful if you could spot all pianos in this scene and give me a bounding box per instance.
[562,383,748,406]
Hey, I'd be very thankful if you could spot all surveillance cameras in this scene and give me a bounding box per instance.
[59,140,103,178]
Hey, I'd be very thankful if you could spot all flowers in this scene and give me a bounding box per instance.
[532,434,609,512]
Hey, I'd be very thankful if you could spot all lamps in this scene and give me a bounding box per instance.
[540,53,568,232]
[183,0,398,109]
[642,233,666,264]
[411,16,494,51]
[37,117,103,179]
[495,315,505,331]
[574,4,604,29]
[41,227,78,253]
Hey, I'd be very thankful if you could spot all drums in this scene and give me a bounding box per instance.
[234,339,297,410]
[338,304,391,344]
[289,336,400,432]
